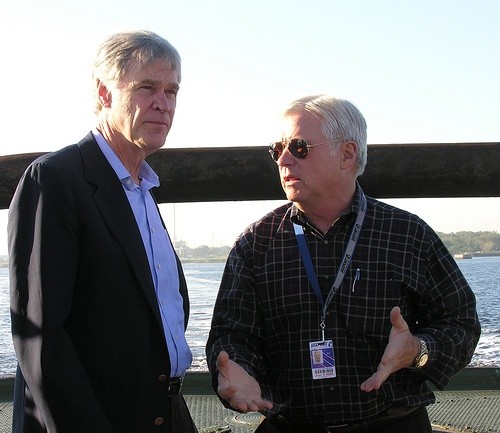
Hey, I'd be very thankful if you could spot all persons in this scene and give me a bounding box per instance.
[7,31,199,433]
[313,351,323,364]
[205,95,481,433]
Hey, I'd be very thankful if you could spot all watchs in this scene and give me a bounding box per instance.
[414,338,430,370]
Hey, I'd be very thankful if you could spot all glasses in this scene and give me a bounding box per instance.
[269,138,345,162]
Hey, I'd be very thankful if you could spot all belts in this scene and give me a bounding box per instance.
[278,406,419,433]
